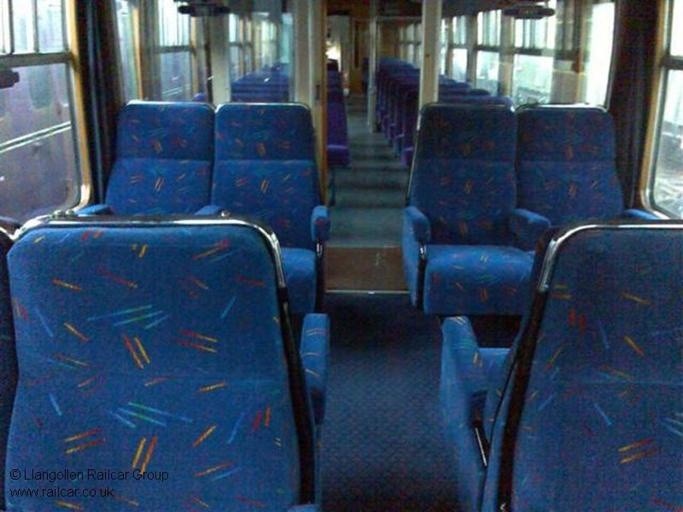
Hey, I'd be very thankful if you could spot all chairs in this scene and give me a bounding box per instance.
[0,52,681,510]
